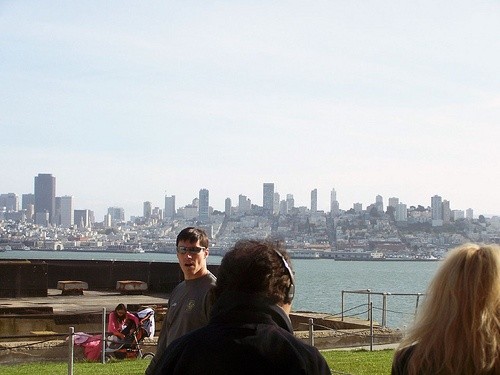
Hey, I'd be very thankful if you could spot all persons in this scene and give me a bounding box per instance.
[105,303,141,341]
[390,242,500,375]
[144,238,333,375]
[119,327,137,344]
[155,227,217,357]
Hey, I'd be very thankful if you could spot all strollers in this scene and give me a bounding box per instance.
[100,309,155,364]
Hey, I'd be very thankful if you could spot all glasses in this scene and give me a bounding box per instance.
[175,246,207,255]
[117,310,125,315]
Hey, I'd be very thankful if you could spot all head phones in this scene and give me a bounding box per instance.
[271,247,295,305]
